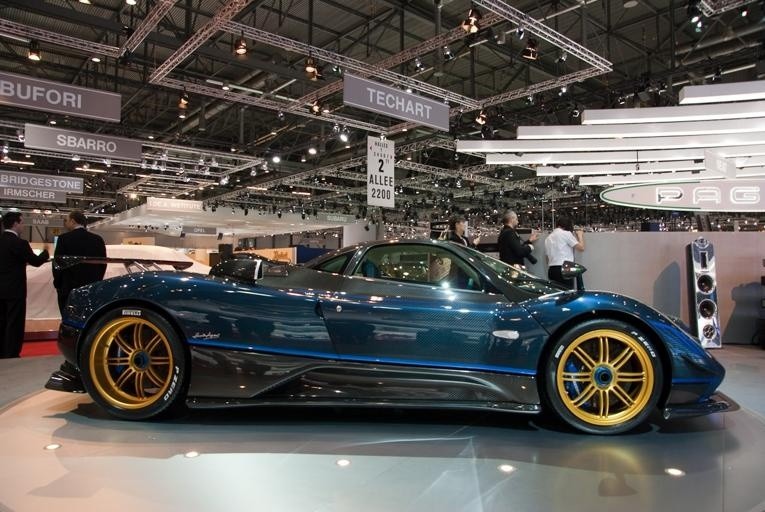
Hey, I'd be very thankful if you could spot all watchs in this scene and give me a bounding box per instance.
[0,212,49,357]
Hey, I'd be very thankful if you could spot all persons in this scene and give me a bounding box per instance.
[446,215,481,289]
[52,211,107,319]
[545,214,585,289]
[497,211,539,267]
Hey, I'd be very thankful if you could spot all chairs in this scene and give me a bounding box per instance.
[361,259,380,280]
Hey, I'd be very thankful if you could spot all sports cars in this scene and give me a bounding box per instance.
[44,239,741,436]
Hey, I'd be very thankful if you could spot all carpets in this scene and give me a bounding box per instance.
[19,339,60,359]
